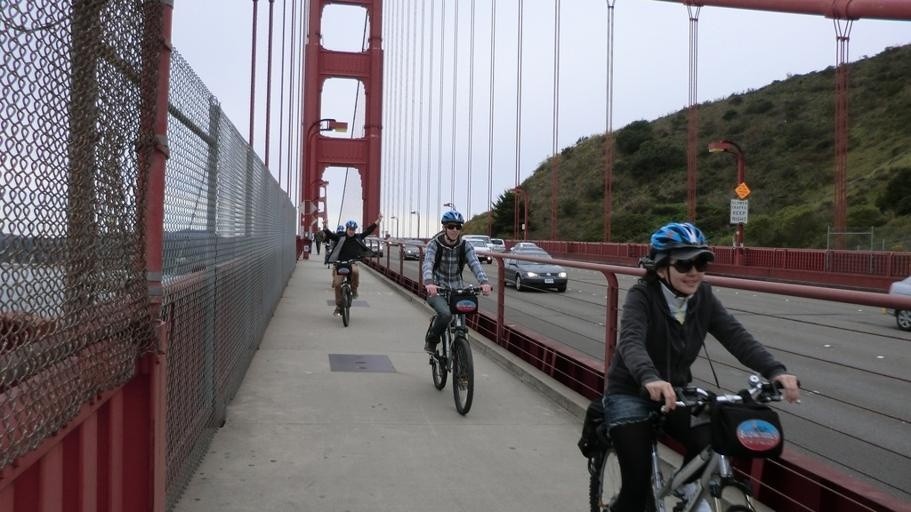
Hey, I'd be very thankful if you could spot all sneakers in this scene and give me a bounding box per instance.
[425,340,438,353]
[352,291,357,297]
[333,308,340,317]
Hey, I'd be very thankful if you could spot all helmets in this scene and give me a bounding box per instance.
[336,226,344,231]
[346,221,358,230]
[648,222,711,250]
[441,212,465,224]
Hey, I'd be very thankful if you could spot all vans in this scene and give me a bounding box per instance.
[462,234,494,250]
[491,238,506,252]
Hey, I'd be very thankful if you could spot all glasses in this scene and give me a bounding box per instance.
[667,260,711,272]
[445,222,460,230]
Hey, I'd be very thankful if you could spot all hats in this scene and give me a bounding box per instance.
[654,248,715,262]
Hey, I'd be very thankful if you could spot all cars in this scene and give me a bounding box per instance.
[402,240,426,260]
[511,242,536,253]
[503,248,567,293]
[887,277,905,327]
[466,239,492,264]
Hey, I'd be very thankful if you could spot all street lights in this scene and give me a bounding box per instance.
[707,139,744,249]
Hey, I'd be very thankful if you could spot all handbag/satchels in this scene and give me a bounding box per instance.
[336,262,351,275]
[449,289,478,315]
[708,401,782,459]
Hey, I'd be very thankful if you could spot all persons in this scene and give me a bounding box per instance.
[603,222,801,512]
[323,213,383,317]
[312,224,344,288]
[422,211,491,354]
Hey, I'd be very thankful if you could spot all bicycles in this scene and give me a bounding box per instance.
[430,283,494,414]
[325,256,362,327]
[577,373,800,512]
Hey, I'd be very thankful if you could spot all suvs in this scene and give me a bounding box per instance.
[363,235,383,256]
